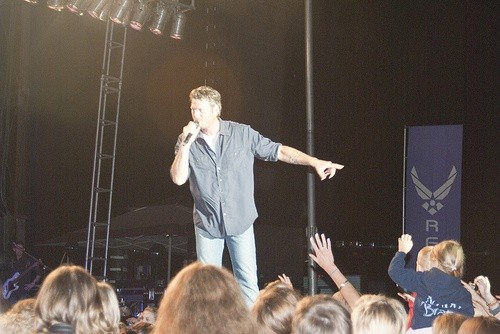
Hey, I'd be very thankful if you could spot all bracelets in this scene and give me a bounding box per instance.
[486,299,497,310]
[338,280,349,291]
[329,268,338,276]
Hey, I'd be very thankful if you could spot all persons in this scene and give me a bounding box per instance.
[0,233,500,334]
[170,86,344,312]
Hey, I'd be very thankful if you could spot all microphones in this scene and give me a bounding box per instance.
[183,121,199,146]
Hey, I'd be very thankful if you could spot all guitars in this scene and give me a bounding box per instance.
[1,258,42,300]
